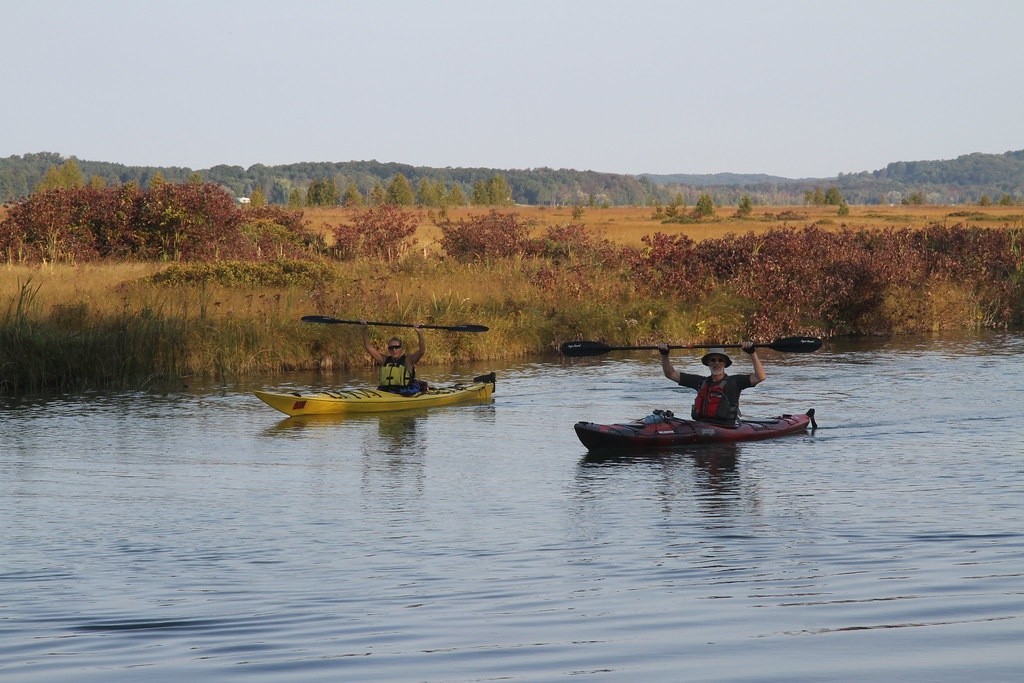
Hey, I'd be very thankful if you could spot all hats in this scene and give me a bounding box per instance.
[701,347,732,368]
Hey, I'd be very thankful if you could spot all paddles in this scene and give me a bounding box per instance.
[560,337,822,357]
[299,314,489,331]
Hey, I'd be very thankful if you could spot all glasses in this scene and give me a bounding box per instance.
[388,344,402,350]
[707,358,724,362]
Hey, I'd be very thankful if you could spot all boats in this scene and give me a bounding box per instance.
[574,408,819,452]
[252,372,497,418]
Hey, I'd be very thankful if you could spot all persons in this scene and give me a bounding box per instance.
[358,318,425,393]
[658,342,766,425]
